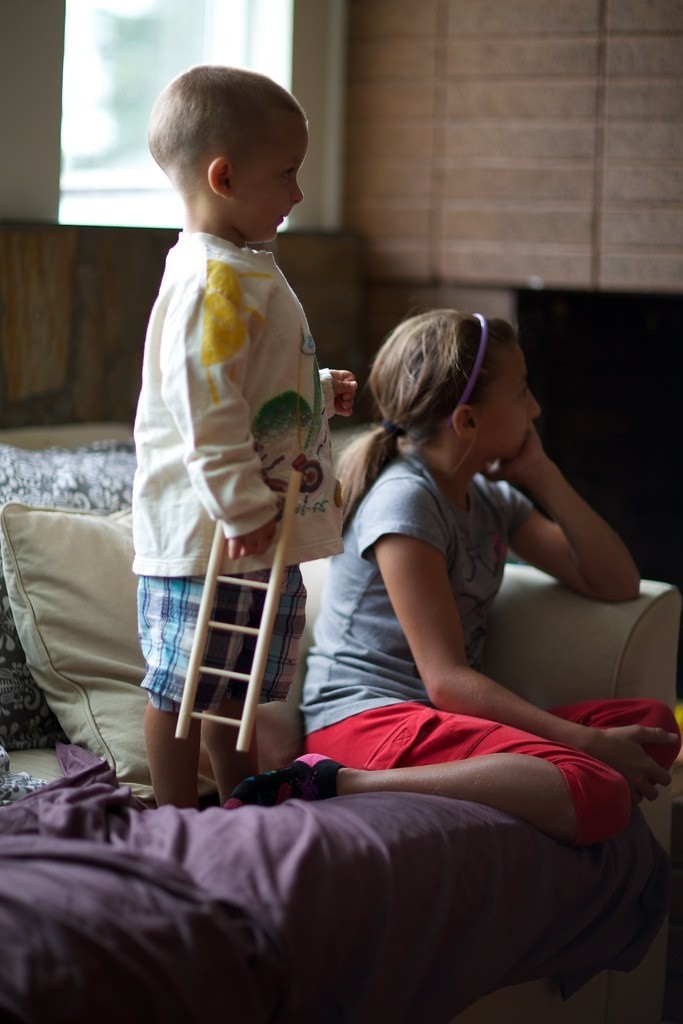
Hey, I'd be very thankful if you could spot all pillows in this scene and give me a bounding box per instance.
[0,427,140,753]
[0,499,330,788]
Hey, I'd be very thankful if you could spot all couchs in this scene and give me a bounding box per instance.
[0,424,683,1024]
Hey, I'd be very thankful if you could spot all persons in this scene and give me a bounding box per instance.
[132,64,356,805]
[224,303,682,849]
